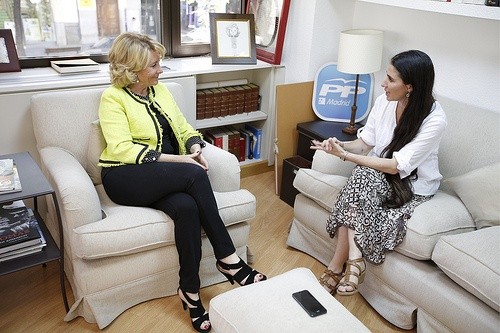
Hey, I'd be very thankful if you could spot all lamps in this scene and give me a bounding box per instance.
[337,29,382,134]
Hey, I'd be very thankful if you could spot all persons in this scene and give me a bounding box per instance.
[310,50,446,295]
[99,33,267,333]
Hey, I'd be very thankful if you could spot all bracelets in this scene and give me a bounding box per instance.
[341,150,348,161]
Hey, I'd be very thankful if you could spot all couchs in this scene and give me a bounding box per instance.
[30,82,256,330]
[286,96,500,332]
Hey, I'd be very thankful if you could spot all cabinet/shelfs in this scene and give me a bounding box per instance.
[196,111,275,177]
[0,151,70,315]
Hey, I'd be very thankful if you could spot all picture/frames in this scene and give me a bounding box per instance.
[208,12,257,64]
[0,29,23,70]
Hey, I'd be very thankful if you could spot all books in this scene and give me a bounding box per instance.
[196,82,263,163]
[0,159,46,261]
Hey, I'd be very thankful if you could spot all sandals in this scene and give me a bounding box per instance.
[319,269,344,295]
[336,258,366,295]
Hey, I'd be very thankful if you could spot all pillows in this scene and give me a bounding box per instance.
[442,163,500,229]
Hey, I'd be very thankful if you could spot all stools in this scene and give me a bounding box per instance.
[206,267,373,333]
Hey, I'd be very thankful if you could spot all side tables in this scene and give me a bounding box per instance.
[296,118,364,161]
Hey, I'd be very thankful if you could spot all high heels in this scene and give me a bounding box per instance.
[177,286,212,333]
[216,256,266,286]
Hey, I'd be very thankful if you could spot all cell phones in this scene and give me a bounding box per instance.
[291,290,327,317]
